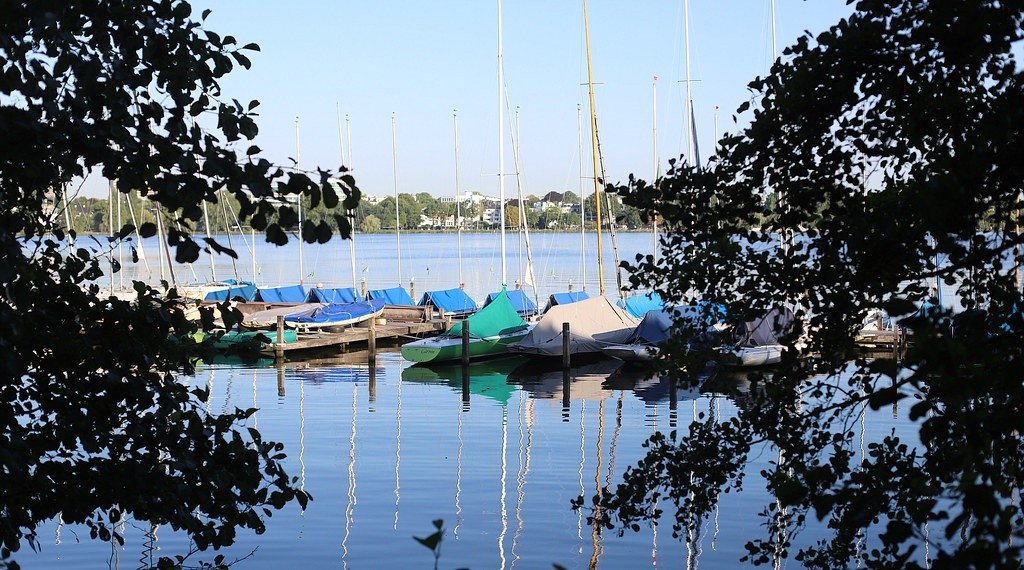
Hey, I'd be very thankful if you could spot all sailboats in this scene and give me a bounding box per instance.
[49,0,1023,370]
